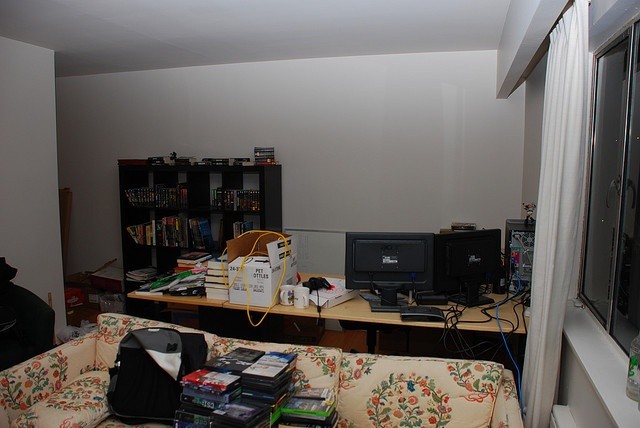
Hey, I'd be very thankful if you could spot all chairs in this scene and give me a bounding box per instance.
[338,319,391,353]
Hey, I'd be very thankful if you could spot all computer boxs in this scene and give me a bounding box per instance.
[504,217,537,302]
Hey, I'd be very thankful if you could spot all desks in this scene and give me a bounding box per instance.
[127,261,529,356]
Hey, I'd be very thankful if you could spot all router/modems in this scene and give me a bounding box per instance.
[450,222,476,230]
[400,305,446,322]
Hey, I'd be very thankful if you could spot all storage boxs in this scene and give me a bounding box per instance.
[225,231,298,307]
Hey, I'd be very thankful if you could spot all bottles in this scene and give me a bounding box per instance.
[625,335,640,402]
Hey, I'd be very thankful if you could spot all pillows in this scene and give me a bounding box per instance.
[10,366,112,427]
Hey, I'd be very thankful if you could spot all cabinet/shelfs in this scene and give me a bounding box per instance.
[118,159,282,315]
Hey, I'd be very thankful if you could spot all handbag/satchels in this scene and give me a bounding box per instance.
[106,326,208,425]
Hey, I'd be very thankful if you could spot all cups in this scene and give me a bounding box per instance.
[294,287,309,308]
[279,285,294,306]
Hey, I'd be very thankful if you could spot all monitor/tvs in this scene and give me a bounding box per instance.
[434,227,504,307]
[344,230,433,313]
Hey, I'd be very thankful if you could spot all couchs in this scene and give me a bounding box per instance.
[0,313,524,427]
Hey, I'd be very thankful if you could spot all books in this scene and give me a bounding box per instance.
[125,186,155,207]
[174,156,196,165]
[232,160,254,167]
[206,255,228,301]
[210,158,229,164]
[135,281,168,294]
[126,267,157,280]
[218,218,223,249]
[212,188,247,210]
[248,189,260,210]
[174,251,212,272]
[149,274,180,292]
[155,183,188,208]
[233,220,253,238]
[148,156,172,166]
[254,147,277,165]
[126,216,214,248]
[174,346,340,426]
[196,278,205,280]
